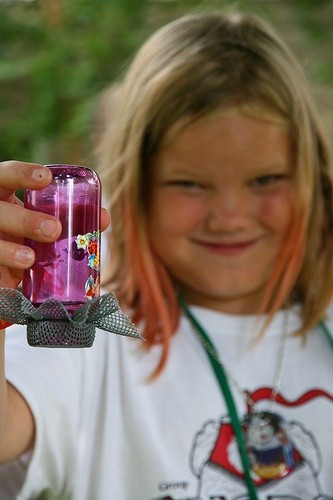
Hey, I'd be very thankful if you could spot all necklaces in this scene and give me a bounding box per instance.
[185,301,289,416]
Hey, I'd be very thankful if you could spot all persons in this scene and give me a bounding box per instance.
[0,11,333,500]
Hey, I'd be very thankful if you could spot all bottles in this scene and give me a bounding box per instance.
[22,164,102,347]
